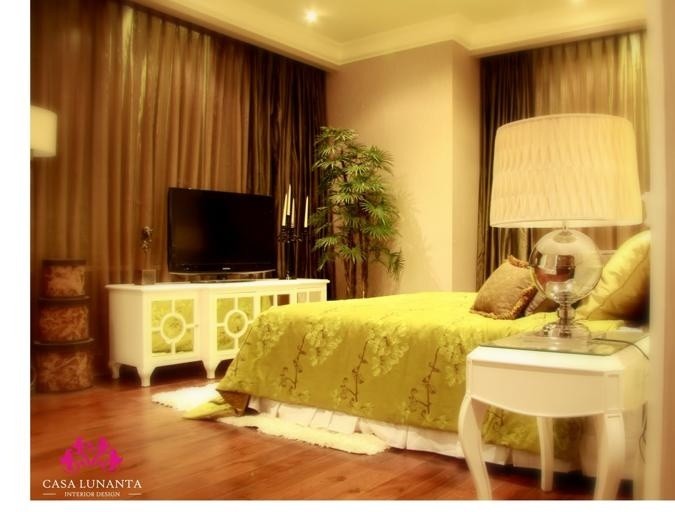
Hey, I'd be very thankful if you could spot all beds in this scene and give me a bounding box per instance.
[458,324,650,500]
[249,289,642,493]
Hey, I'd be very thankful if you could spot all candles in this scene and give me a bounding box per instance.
[281,183,310,230]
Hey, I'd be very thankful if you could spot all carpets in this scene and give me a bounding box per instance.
[151,381,395,457]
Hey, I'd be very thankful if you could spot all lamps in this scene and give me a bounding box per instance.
[486,113,646,339]
[30,104,59,163]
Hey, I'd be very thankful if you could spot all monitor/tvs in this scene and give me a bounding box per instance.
[166,187,278,283]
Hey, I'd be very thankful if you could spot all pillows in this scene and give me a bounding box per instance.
[468,230,649,321]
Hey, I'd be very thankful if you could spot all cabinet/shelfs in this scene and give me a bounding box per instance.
[104,277,330,387]
